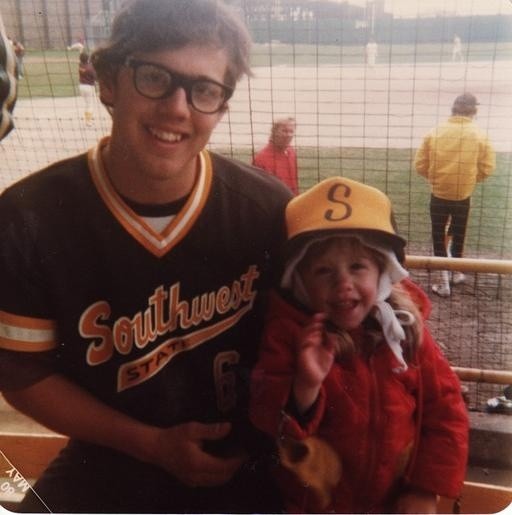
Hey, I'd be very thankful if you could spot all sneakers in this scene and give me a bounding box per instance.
[486,394,512,412]
[450,272,466,284]
[432,283,452,298]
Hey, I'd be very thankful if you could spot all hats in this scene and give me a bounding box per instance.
[285,176,407,253]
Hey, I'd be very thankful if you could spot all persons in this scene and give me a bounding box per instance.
[12,37,27,79]
[0,0,299,513]
[413,90,497,298]
[451,34,466,63]
[484,383,511,416]
[248,174,470,512]
[365,33,379,70]
[79,52,99,127]
[248,115,301,198]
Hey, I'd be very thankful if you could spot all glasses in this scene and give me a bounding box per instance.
[120,56,233,115]
